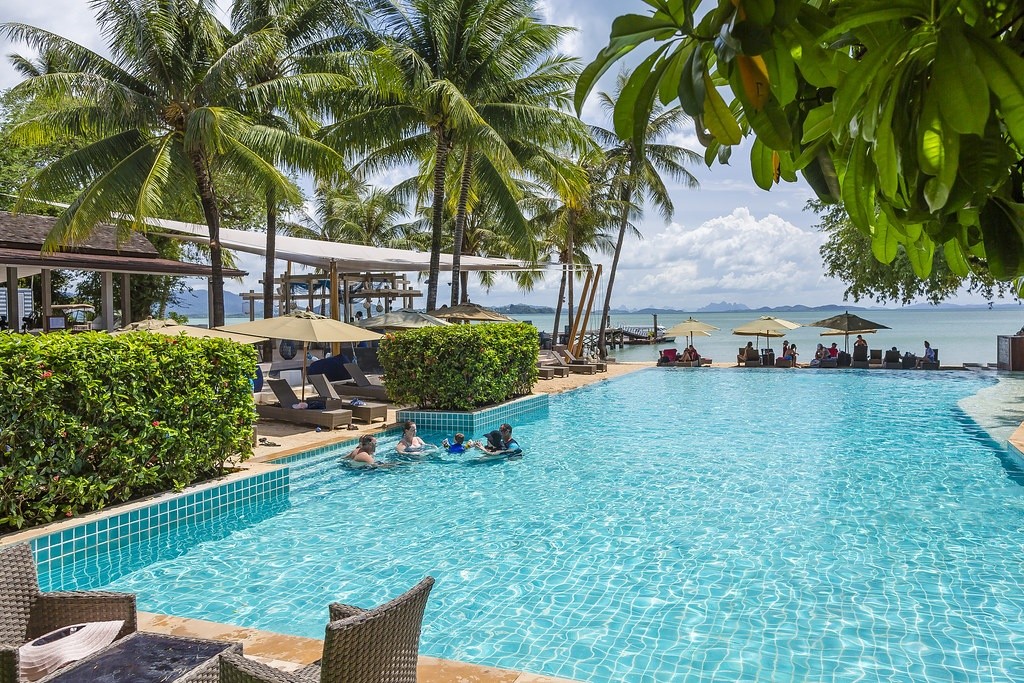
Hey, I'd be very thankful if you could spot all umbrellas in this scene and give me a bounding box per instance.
[661,311,892,354]
[105,303,513,403]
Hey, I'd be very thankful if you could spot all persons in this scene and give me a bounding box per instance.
[682,345,697,362]
[496,423,521,455]
[356,311,363,321]
[854,335,867,347]
[476,430,506,456]
[0,316,9,331]
[883,347,903,364]
[744,342,753,360]
[828,343,838,358]
[785,344,799,368]
[353,435,377,463]
[912,341,935,369]
[346,435,365,459]
[443,433,474,453]
[816,344,830,361]
[783,340,788,357]
[396,422,438,456]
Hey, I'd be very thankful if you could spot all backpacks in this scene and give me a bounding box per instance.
[307,398,326,409]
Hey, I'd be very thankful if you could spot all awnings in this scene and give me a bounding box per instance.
[0,195,604,356]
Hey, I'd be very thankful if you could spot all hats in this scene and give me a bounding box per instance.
[482,430,503,448]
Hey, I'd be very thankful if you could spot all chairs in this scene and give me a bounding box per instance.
[658,348,712,366]
[334,363,391,401]
[739,348,793,366]
[307,374,388,424]
[535,349,607,381]
[218,576,436,683]
[256,378,352,431]
[821,349,940,369]
[0,542,138,683]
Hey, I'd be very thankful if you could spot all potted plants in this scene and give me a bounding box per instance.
[836,351,851,366]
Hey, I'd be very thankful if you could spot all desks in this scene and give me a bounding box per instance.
[306,397,342,410]
[34,631,242,683]
